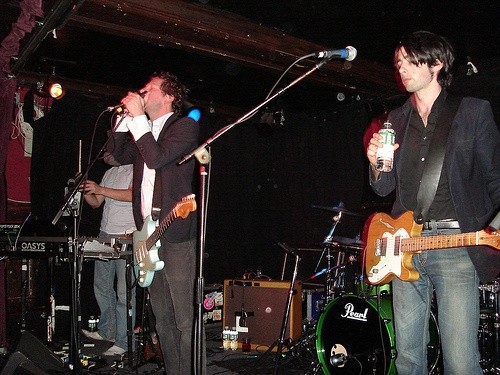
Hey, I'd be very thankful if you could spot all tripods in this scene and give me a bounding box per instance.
[51,112,163,375]
[246,214,500,375]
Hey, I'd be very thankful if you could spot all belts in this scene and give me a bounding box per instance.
[422,218,460,230]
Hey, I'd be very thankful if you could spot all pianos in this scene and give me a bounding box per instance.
[0,233,148,371]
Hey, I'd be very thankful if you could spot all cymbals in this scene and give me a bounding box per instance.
[311,205,364,218]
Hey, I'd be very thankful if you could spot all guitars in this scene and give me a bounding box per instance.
[365,209,500,286]
[133,193,197,288]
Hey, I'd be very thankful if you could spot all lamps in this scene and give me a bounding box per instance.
[33,79,63,98]
[180,100,203,124]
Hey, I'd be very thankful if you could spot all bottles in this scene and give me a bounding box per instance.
[230,327,238,350]
[374,120,395,173]
[97,316,100,324]
[88,316,96,332]
[222,326,231,351]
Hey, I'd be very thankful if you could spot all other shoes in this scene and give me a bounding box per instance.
[102,345,127,356]
[82,329,116,342]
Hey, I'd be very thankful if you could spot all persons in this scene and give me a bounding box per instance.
[362,31,500,375]
[83,71,202,375]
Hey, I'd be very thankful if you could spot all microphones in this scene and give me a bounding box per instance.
[116,93,144,113]
[314,45,357,61]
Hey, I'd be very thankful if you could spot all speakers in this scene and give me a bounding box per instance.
[223,279,303,352]
[0,332,66,375]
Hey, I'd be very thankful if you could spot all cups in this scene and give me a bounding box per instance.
[242,337,252,355]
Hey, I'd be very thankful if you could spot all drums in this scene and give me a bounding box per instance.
[315,294,440,375]
[477,282,497,327]
[339,245,392,299]
